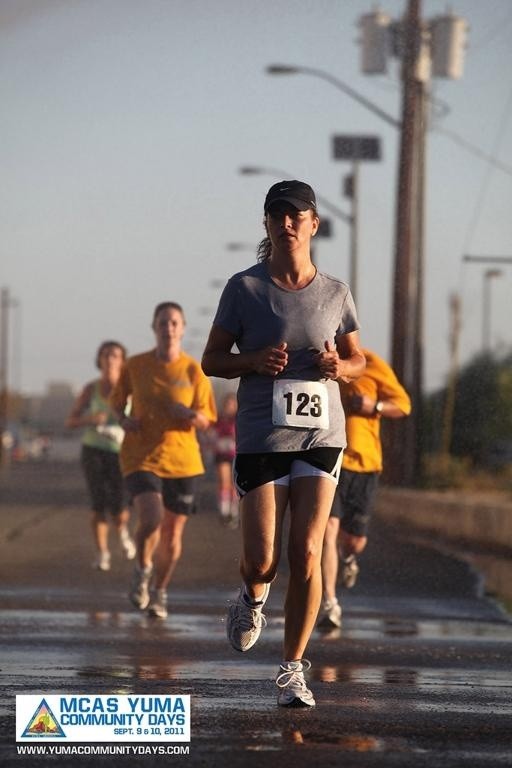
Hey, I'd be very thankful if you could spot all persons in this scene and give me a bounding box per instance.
[200,179,368,709]
[62,341,136,573]
[112,299,219,620]
[314,346,415,629]
[204,393,240,531]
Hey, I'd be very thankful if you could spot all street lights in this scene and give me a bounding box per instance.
[266,65,431,487]
[240,157,361,329]
[176,239,259,377]
[479,265,503,353]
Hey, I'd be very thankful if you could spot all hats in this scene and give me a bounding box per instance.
[261,178,320,216]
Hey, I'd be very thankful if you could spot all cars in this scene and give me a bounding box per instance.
[10,436,50,467]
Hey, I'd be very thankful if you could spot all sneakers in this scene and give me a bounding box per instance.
[276,656,317,709]
[315,592,343,629]
[128,566,152,610]
[149,590,168,618]
[92,548,111,572]
[119,537,137,561]
[226,579,270,654]
[338,550,361,590]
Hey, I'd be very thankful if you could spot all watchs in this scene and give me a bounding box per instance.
[371,398,383,420]
